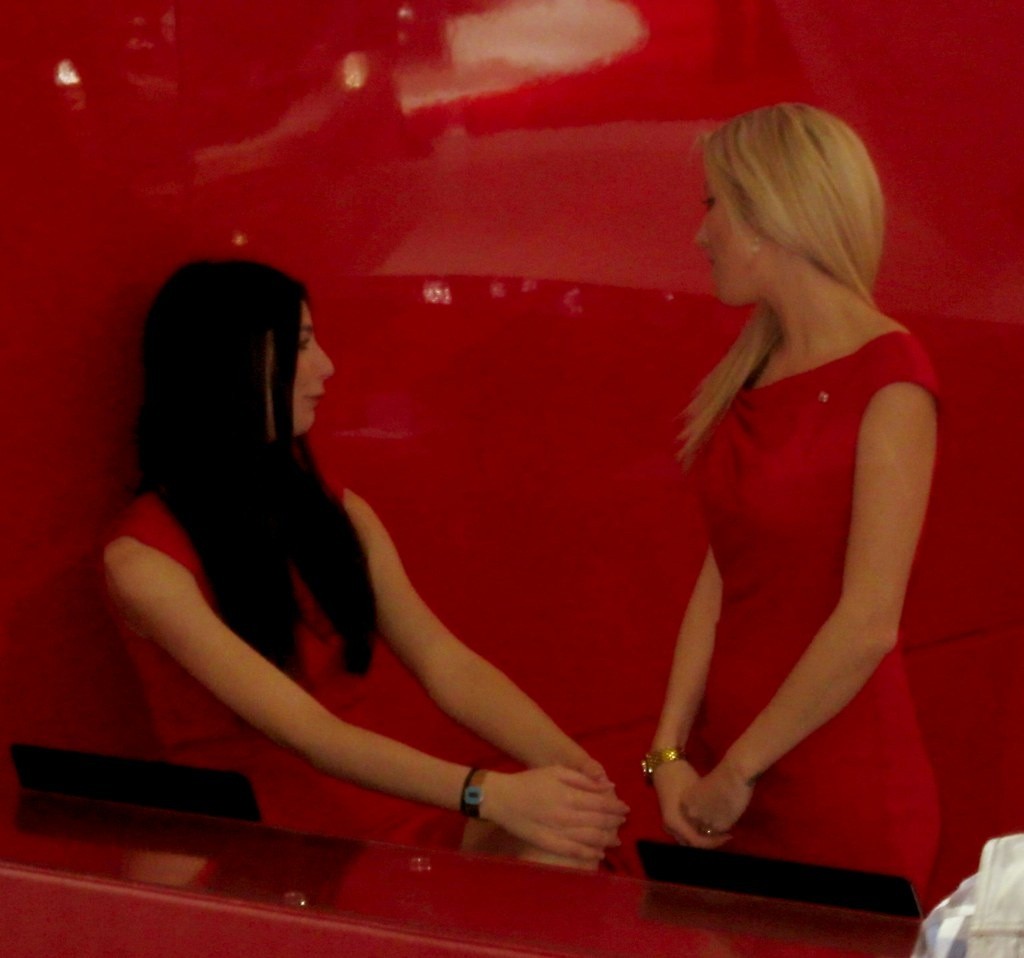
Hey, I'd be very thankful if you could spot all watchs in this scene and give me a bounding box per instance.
[640,745,685,791]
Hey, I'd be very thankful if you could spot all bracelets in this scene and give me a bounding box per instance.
[460,767,488,821]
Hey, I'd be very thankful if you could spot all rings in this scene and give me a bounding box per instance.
[698,825,713,838]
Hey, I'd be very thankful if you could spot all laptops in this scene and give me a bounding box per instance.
[9,742,259,823]
[632,839,925,919]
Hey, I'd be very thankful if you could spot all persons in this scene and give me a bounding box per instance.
[631,107,942,909]
[96,259,629,872]
